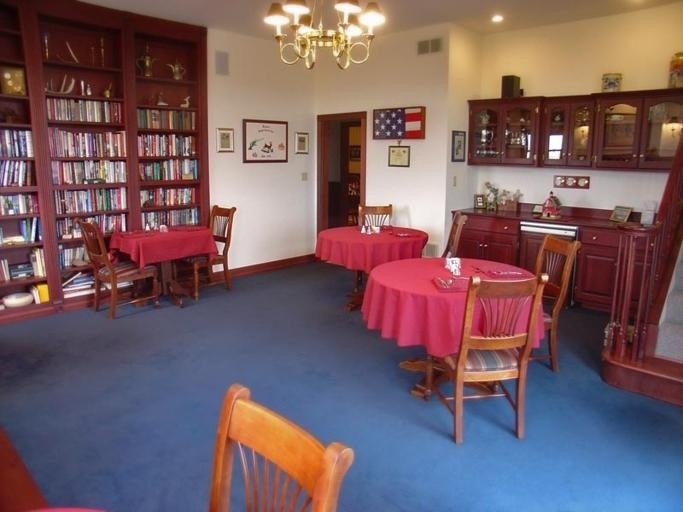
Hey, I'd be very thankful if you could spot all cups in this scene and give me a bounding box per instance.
[639,200,656,226]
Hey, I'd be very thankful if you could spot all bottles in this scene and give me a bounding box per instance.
[475,144,498,156]
[504,128,527,145]
[144,223,150,230]
[445,257,461,276]
[360,225,370,234]
[152,220,158,231]
[479,128,493,144]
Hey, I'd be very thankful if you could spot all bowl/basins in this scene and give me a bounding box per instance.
[2,292,34,309]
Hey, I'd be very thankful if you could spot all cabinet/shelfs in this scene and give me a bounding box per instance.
[128,9,211,296]
[0,0,58,325]
[539,94,594,171]
[574,219,657,319]
[468,95,543,166]
[23,2,140,309]
[451,208,519,267]
[593,87,682,171]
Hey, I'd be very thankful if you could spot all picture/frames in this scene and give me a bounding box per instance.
[608,206,632,222]
[242,118,289,163]
[292,131,309,154]
[473,193,486,209]
[216,127,234,153]
[451,129,465,162]
[387,145,410,168]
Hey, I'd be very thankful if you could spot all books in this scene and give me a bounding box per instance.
[139,109,198,230]
[0,128,47,282]
[48,99,133,299]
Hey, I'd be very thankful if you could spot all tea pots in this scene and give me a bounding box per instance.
[136,42,159,77]
[162,58,186,81]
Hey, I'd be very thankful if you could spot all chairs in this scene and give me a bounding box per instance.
[356,203,391,228]
[439,210,467,257]
[432,273,549,447]
[526,235,579,373]
[172,205,235,300]
[77,220,161,318]
[208,384,352,511]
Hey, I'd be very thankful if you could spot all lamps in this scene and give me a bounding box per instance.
[263,0,385,68]
